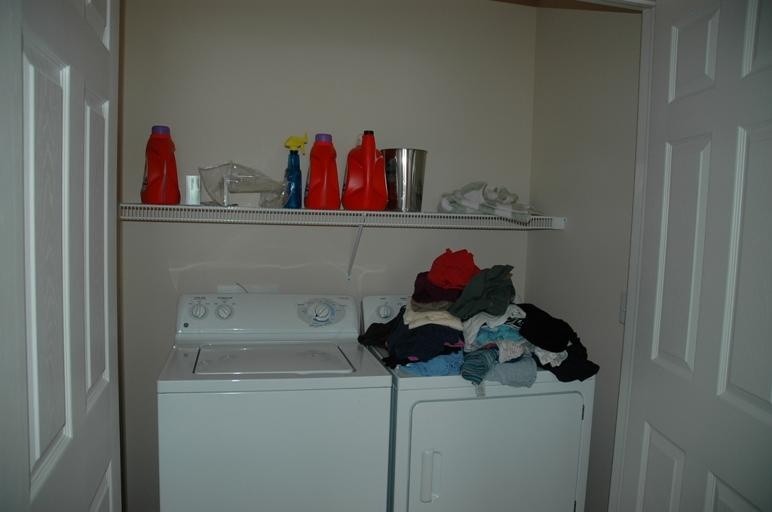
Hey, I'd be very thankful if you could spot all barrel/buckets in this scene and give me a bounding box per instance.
[381,147,429,213]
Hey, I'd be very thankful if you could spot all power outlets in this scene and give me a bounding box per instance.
[619,291,627,326]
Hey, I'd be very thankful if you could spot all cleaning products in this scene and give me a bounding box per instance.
[283,150,302,207]
[306,134,341,210]
[141,126,179,203]
[342,130,388,210]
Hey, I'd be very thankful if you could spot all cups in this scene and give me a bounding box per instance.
[185,175,199,205]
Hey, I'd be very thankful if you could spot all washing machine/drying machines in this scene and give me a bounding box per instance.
[361,292,597,512]
[152,291,393,512]
